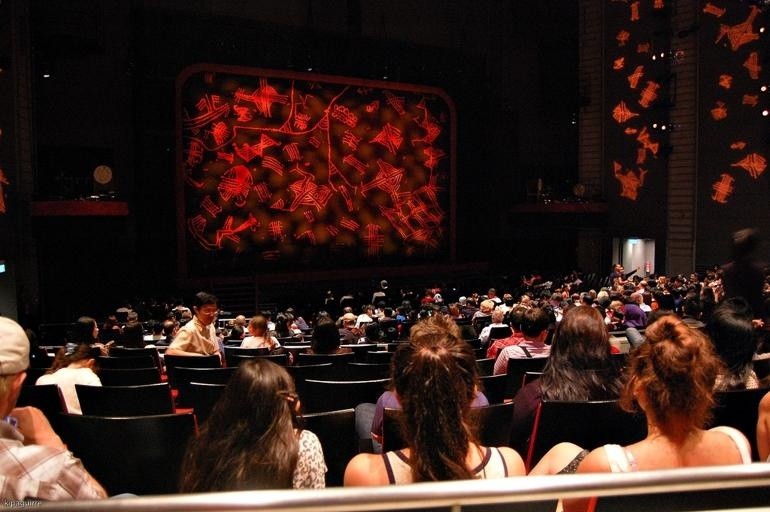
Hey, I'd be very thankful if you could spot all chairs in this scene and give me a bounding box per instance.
[164,355,221,389]
[298,352,356,379]
[225,340,242,346]
[300,328,312,335]
[475,320,492,336]
[75,384,175,418]
[525,399,649,477]
[461,325,474,340]
[108,347,162,374]
[544,329,554,345]
[754,327,770,340]
[48,412,198,496]
[475,358,496,376]
[24,367,52,386]
[229,355,288,366]
[190,382,228,427]
[224,347,269,367]
[96,368,161,386]
[363,351,396,363]
[158,348,167,354]
[387,343,399,352]
[144,340,158,345]
[607,353,626,373]
[36,346,49,367]
[339,345,377,363]
[753,358,770,380]
[95,355,156,368]
[16,384,67,413]
[284,363,335,392]
[346,362,394,381]
[400,322,418,341]
[707,388,769,462]
[382,401,515,453]
[381,319,401,342]
[175,367,239,408]
[473,315,492,329]
[608,331,627,337]
[276,336,302,346]
[102,329,120,344]
[464,338,481,350]
[472,349,487,359]
[284,340,312,346]
[638,329,645,335]
[294,407,358,487]
[301,378,392,412]
[496,347,504,361]
[479,373,508,406]
[283,346,312,361]
[339,339,353,344]
[523,372,544,387]
[483,327,512,349]
[506,357,550,399]
[359,322,373,328]
[454,319,473,326]
[54,345,102,358]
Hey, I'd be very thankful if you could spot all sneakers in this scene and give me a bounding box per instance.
[0,316,32,378]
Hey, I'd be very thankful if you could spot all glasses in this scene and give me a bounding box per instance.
[197,309,218,316]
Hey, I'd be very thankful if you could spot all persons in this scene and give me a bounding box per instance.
[325,290,335,310]
[144,319,156,335]
[721,231,763,296]
[173,309,182,321]
[432,283,442,295]
[165,292,222,366]
[681,300,721,359]
[355,305,375,329]
[366,314,380,327]
[229,318,243,340]
[448,302,456,310]
[432,303,440,312]
[597,291,611,309]
[239,316,281,349]
[459,296,467,306]
[674,293,682,314]
[399,287,406,297]
[317,311,334,325]
[478,311,509,347]
[499,294,513,314]
[396,306,406,321]
[162,315,176,337]
[420,289,436,308]
[271,314,295,338]
[260,310,276,330]
[124,322,155,348]
[302,322,352,355]
[116,304,133,315]
[35,342,103,415]
[610,263,721,293]
[215,329,226,363]
[489,288,502,303]
[609,292,622,300]
[452,311,462,319]
[343,318,361,345]
[461,300,473,319]
[420,310,429,319]
[102,315,123,336]
[154,324,175,346]
[472,300,494,325]
[375,301,385,317]
[519,264,596,320]
[284,312,301,335]
[0,316,107,507]
[408,310,418,322]
[704,296,760,392]
[343,333,527,487]
[391,304,398,317]
[764,266,770,295]
[642,293,652,313]
[173,299,185,311]
[177,358,329,494]
[143,322,167,340]
[354,312,489,456]
[235,315,246,340]
[336,307,357,326]
[433,291,443,306]
[380,280,392,301]
[471,293,479,306]
[603,300,625,331]
[17,316,37,350]
[378,308,396,326]
[214,309,220,329]
[650,294,659,312]
[182,310,191,318]
[401,289,418,309]
[493,308,552,375]
[486,307,528,360]
[286,307,308,329]
[622,296,648,328]
[65,317,110,355]
[525,314,752,478]
[601,287,610,293]
[125,312,144,335]
[509,307,626,463]
[658,293,677,314]
[757,391,770,463]
